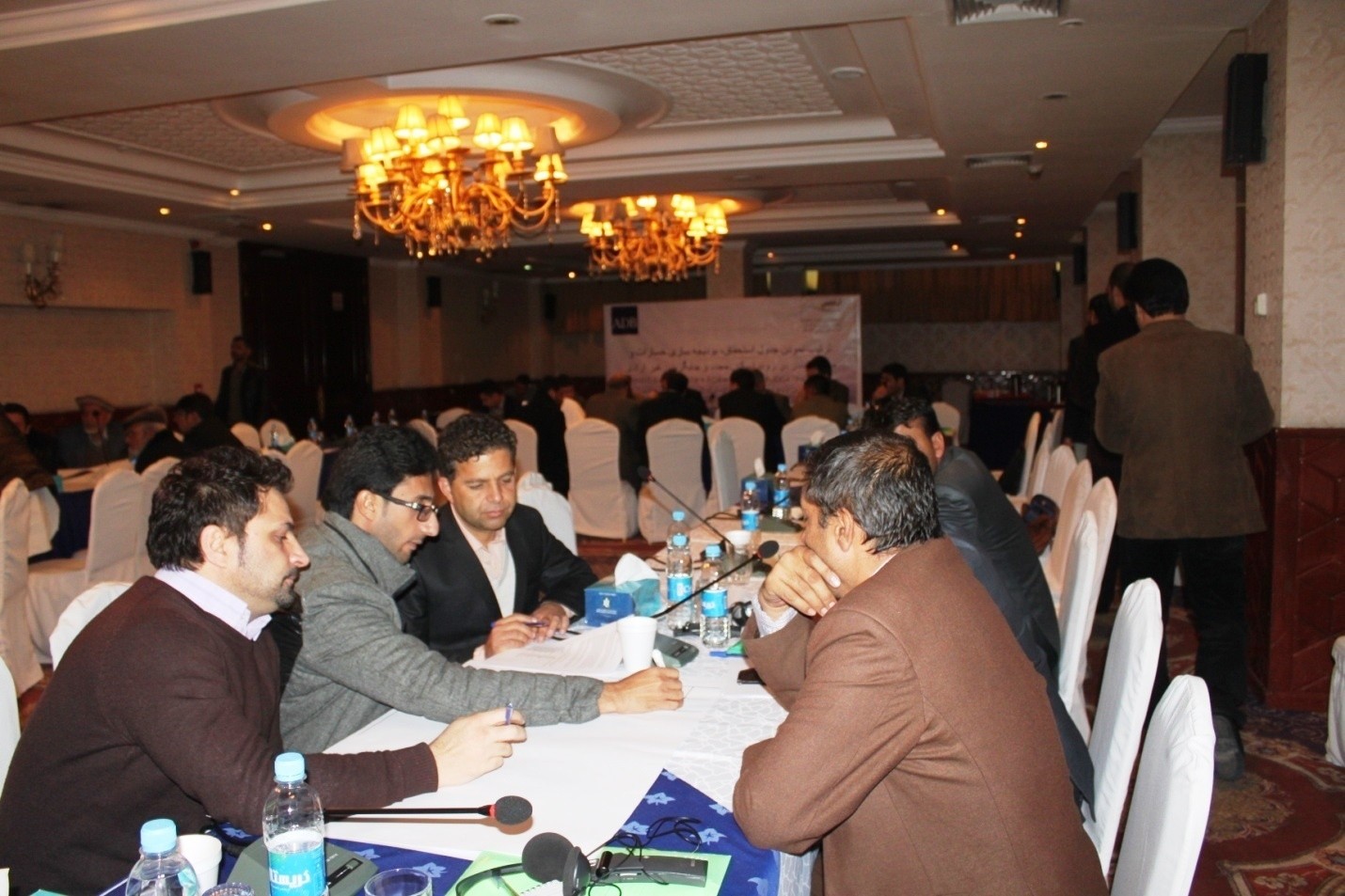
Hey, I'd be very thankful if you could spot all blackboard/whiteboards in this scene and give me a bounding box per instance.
[601,296,864,410]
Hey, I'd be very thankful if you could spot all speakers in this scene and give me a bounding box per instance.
[540,283,558,319]
[1114,191,1138,254]
[427,275,444,309]
[1050,267,1058,303]
[1074,232,1086,288]
[190,249,212,293]
[1223,52,1266,166]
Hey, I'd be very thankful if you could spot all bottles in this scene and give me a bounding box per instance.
[699,546,732,646]
[262,753,329,895]
[740,479,762,551]
[126,818,200,896]
[665,509,694,631]
[128,448,138,467]
[371,412,380,428]
[775,465,791,520]
[308,418,319,441]
[270,424,279,449]
[344,415,355,439]
[388,410,395,426]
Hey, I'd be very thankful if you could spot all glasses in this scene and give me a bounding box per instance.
[81,409,103,418]
[376,492,439,522]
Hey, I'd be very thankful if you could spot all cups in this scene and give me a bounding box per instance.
[175,832,223,896]
[618,616,658,672]
[203,883,253,895]
[364,867,433,896]
[726,530,751,554]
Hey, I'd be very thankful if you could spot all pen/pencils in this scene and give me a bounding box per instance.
[490,621,551,628]
[504,702,515,725]
[651,649,667,669]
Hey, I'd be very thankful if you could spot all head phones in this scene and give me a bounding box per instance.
[457,835,591,896]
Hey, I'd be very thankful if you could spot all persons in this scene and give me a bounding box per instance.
[276,420,686,759]
[853,393,1061,665]
[851,361,930,449]
[740,430,1109,895]
[945,540,1095,826]
[436,365,787,488]
[116,403,188,471]
[788,376,848,432]
[788,354,852,403]
[395,410,602,672]
[1083,259,1152,490]
[63,391,133,467]
[173,393,247,460]
[1,401,34,448]
[1,445,526,895]
[1093,256,1272,781]
[213,333,273,427]
[1061,292,1116,436]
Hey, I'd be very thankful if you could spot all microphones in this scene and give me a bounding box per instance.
[636,466,732,548]
[646,540,780,619]
[313,794,532,827]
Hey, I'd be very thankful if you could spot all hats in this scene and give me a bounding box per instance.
[75,393,115,414]
[120,403,168,429]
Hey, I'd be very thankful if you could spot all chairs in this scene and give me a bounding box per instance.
[0,393,1218,894]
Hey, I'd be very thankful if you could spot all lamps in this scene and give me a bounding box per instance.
[580,192,730,283]
[337,92,577,260]
[22,230,65,311]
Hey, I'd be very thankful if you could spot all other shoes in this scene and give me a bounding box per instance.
[1213,713,1244,783]
